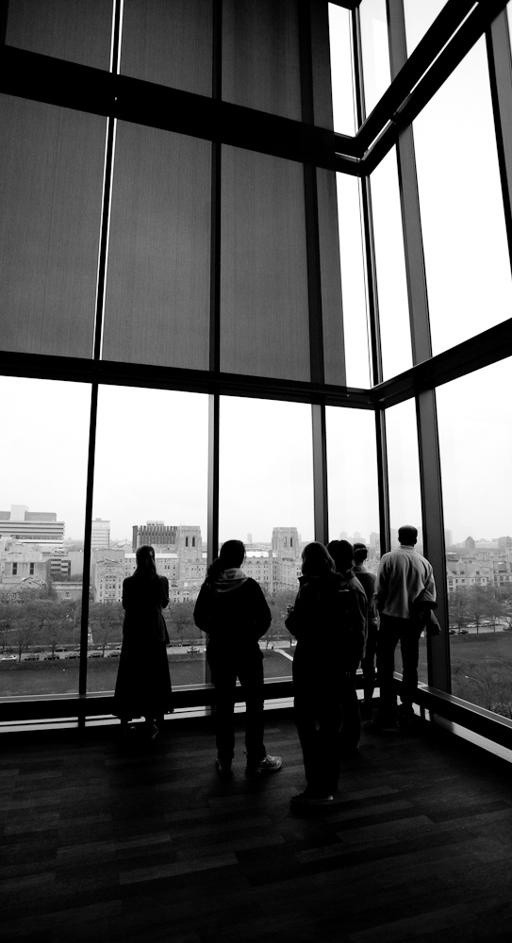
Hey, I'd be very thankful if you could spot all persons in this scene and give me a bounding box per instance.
[194,540,284,776]
[371,526,434,732]
[109,546,174,739]
[353,544,382,710]
[287,542,361,808]
[328,539,371,749]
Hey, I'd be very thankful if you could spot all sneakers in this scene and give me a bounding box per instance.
[291,788,337,808]
[248,753,280,774]
